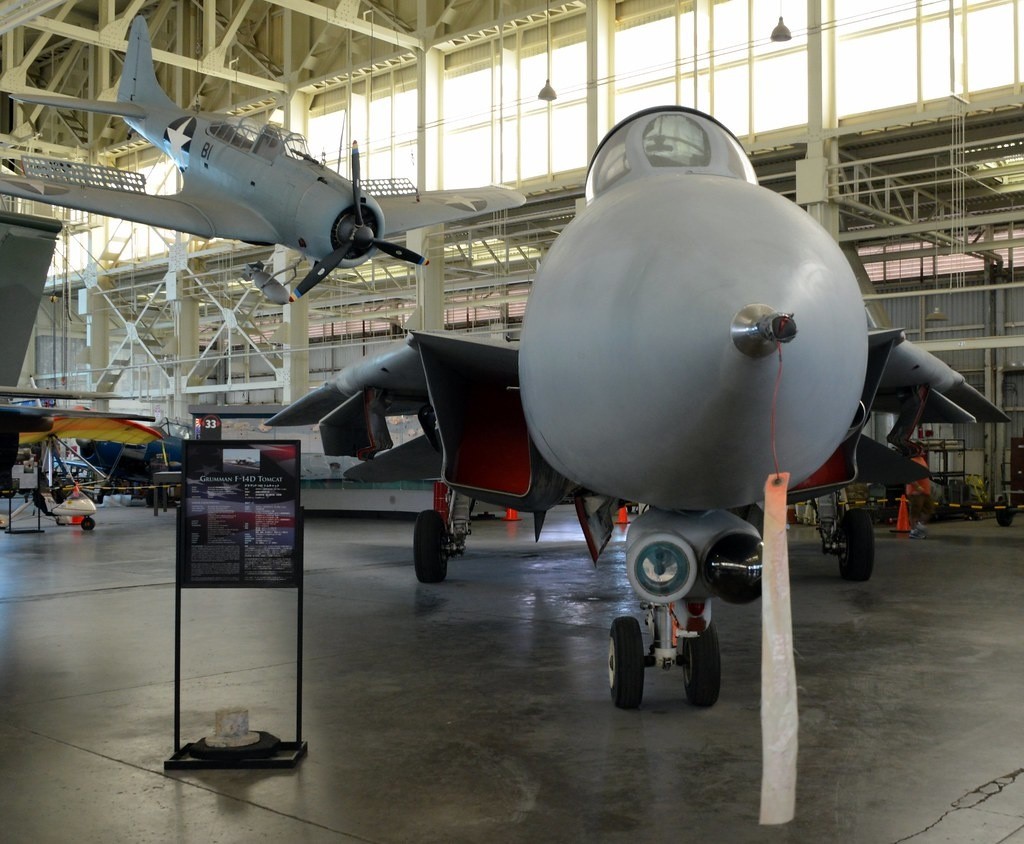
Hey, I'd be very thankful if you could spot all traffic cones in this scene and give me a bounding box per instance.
[68,485,86,525]
[613,503,631,525]
[889,493,913,535]
[500,507,524,522]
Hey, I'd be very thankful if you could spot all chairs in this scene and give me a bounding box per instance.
[249,124,287,163]
[51,486,67,504]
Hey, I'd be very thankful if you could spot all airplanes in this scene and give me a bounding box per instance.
[260,105,1018,713]
[1,13,528,305]
[0,382,192,531]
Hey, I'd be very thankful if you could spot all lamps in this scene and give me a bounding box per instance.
[925,153,948,321]
[538,0,558,102]
[770,0,792,42]
[48,255,60,303]
